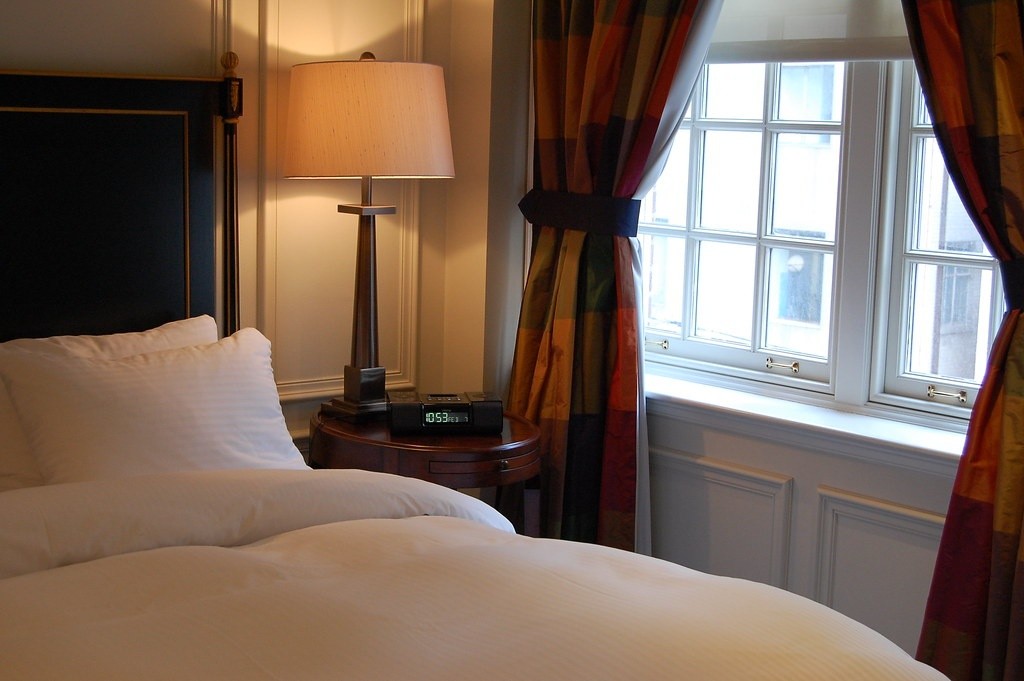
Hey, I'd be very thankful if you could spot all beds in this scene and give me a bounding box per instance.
[0,68,953,681]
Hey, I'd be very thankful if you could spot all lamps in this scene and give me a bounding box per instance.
[282,50,458,422]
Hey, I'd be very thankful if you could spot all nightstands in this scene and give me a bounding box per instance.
[308,410,553,538]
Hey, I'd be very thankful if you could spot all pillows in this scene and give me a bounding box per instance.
[0,326,311,483]
[0,314,217,490]
